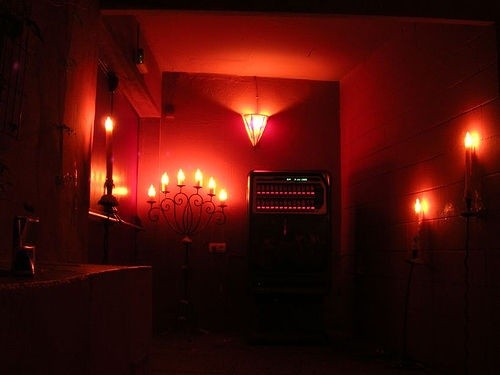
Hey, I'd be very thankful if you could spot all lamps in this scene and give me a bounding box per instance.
[465,131,481,204]
[105,115,115,193]
[241,111,271,148]
[415,195,428,246]
[147,166,230,244]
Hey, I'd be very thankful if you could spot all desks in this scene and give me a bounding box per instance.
[0,261,154,375]
